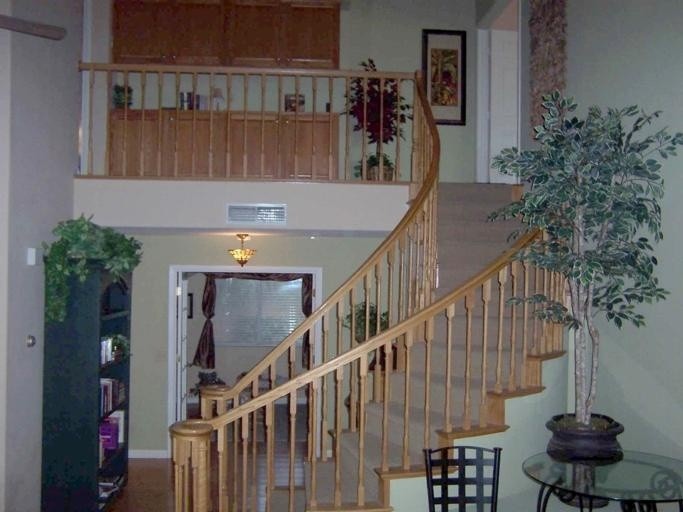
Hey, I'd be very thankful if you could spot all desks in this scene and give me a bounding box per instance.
[521,449,681,512]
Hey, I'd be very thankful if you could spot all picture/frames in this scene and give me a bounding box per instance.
[421,28,467,126]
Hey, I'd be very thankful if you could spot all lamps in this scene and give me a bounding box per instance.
[226,234,257,269]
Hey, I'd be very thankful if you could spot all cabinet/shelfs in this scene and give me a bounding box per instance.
[225,0,340,71]
[225,110,340,183]
[110,0,224,67]
[42,253,130,512]
[109,108,228,177]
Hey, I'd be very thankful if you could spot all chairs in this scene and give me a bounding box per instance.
[422,444,504,512]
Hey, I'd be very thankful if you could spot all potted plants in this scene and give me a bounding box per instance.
[336,57,415,181]
[480,86,683,466]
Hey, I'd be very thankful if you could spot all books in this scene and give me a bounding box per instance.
[99,410,124,468]
[100,378,125,416]
